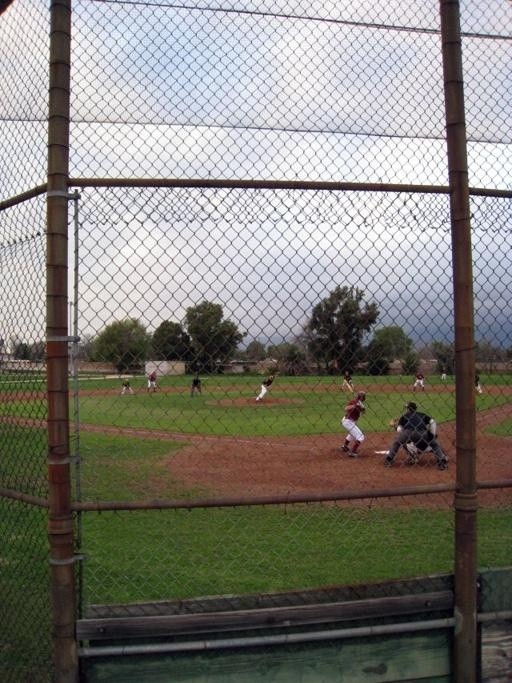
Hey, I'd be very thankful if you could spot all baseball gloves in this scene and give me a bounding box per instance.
[389,418,399,427]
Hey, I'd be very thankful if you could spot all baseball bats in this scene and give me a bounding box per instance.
[344,380,364,410]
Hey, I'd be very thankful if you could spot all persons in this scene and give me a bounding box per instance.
[256,373,275,403]
[384,401,448,470]
[413,371,424,391]
[390,416,448,464]
[441,365,446,379]
[340,390,366,456]
[342,370,354,391]
[148,370,157,391]
[121,378,134,395]
[474,374,482,393]
[191,374,202,397]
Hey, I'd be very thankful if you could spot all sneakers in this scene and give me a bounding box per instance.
[341,446,449,470]
